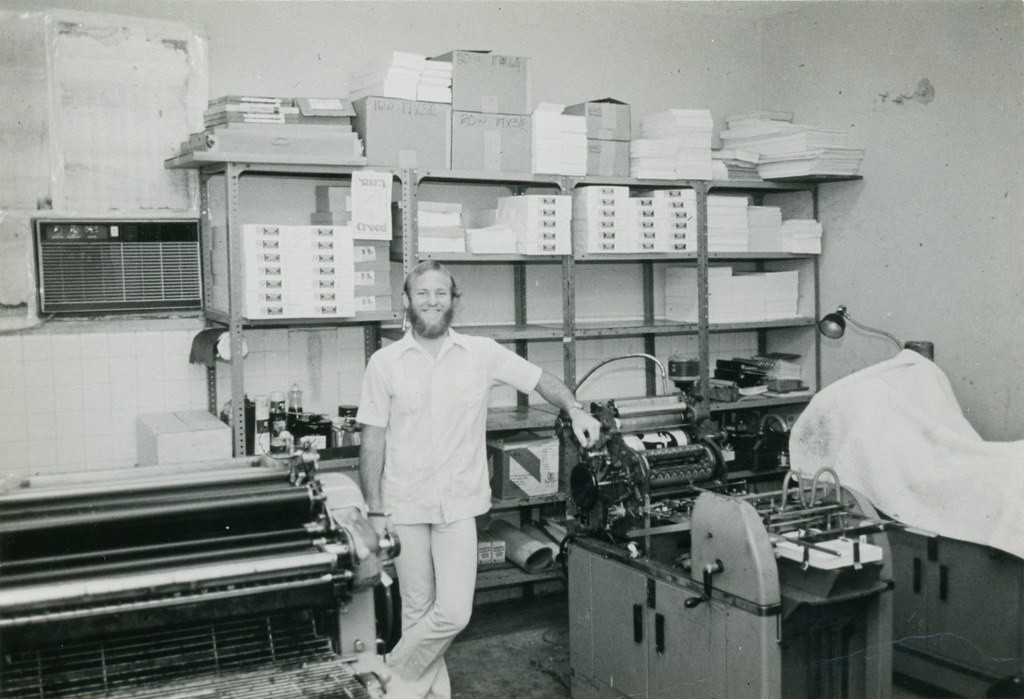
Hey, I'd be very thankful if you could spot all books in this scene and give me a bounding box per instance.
[204,93,302,129]
[347,51,454,105]
[630,105,866,186]
[534,112,592,175]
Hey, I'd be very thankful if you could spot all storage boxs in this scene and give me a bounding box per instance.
[477,531,505,562]
[137,411,235,467]
[212,48,821,323]
[484,434,561,500]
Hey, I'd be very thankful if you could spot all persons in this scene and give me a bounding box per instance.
[359,262,600,699]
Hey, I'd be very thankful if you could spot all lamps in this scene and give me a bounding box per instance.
[820,306,933,362]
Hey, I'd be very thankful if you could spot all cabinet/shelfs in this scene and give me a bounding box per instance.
[564,527,1024,699]
[199,163,821,595]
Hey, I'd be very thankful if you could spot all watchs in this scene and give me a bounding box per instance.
[567,402,584,415]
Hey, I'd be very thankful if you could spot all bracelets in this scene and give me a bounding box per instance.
[366,512,392,520]
